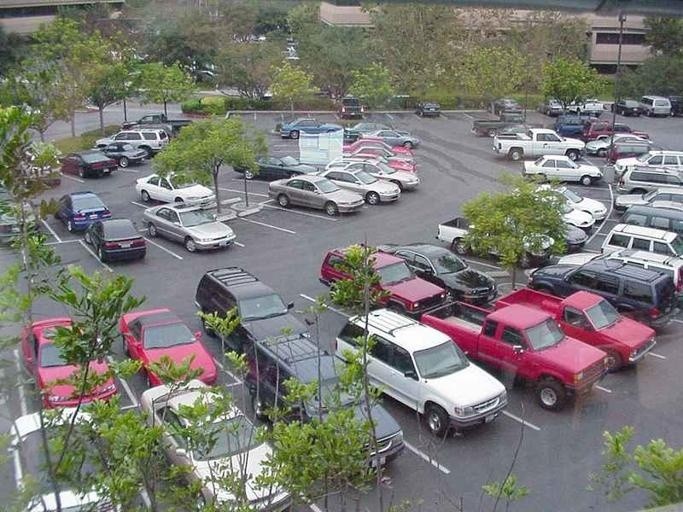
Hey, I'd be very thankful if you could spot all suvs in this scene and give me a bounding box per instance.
[528,263,679,330]
[338,97,365,120]
[194,267,310,356]
[557,250,682,313]
[95,123,179,160]
[335,307,507,437]
[241,332,405,475]
[318,242,446,318]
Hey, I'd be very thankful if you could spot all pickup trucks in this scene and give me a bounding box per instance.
[419,299,609,409]
[120,113,193,131]
[435,214,556,270]
[490,127,587,162]
[496,286,656,371]
[471,113,544,138]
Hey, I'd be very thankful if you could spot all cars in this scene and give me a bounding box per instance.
[83,218,147,263]
[98,141,150,169]
[139,377,297,510]
[355,129,420,150]
[416,101,442,118]
[267,175,366,216]
[20,316,117,409]
[180,64,213,82]
[343,122,392,142]
[54,190,113,234]
[536,96,681,267]
[9,407,152,512]
[521,154,605,187]
[121,308,216,389]
[373,240,497,306]
[1,202,40,244]
[493,98,523,116]
[538,194,597,231]
[560,224,590,251]
[142,201,237,254]
[231,152,319,181]
[61,150,120,178]
[279,117,343,139]
[542,184,608,223]
[134,172,216,205]
[308,137,422,206]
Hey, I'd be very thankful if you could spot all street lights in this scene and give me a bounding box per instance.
[605,8,626,167]
